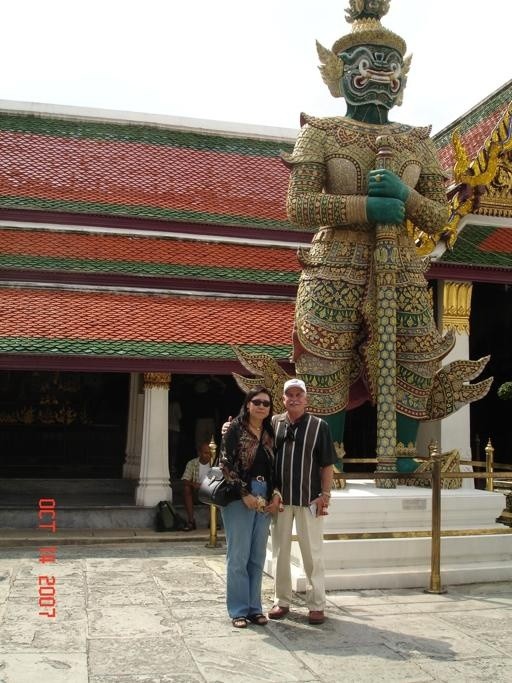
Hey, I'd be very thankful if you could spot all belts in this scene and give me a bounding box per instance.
[251,476,268,481]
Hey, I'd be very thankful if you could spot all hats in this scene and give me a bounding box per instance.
[284,379,305,393]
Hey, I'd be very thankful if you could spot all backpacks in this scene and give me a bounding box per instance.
[154,501,178,531]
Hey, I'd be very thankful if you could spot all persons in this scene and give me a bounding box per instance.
[219,379,339,627]
[181,442,221,531]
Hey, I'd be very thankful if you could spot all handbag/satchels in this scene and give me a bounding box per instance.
[199,467,242,507]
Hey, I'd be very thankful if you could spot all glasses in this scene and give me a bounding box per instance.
[249,399,270,407]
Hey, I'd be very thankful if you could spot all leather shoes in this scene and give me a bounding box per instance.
[309,611,324,624]
[268,605,289,619]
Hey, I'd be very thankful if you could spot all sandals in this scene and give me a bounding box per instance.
[208,523,221,530]
[232,613,268,628]
[183,519,196,531]
